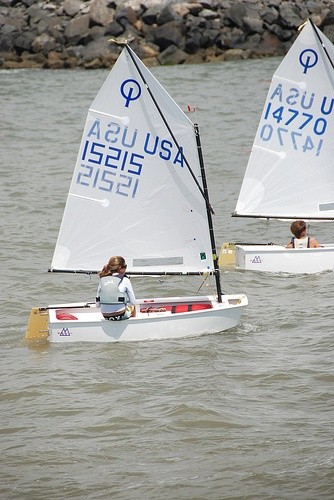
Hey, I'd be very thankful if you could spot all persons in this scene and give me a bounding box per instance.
[285,221,319,248]
[95,256,137,321]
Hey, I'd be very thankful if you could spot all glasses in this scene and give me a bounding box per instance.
[121,265,127,269]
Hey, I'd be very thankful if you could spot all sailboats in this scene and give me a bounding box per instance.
[216,17,334,275]
[25,37,250,345]
